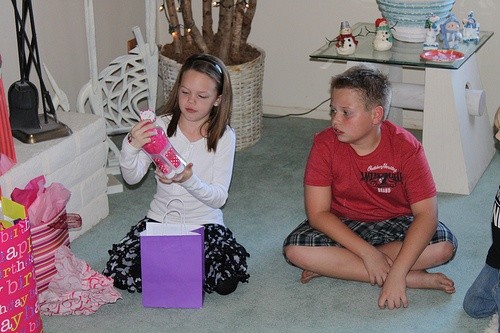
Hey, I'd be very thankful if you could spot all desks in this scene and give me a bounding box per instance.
[308,20,497,196]
[0,108,110,243]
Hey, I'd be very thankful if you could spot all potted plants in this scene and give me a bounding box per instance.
[158,0,265,153]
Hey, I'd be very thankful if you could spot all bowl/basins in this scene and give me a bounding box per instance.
[376,0,455,43]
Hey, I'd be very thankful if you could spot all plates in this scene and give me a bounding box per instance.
[419,50,465,62]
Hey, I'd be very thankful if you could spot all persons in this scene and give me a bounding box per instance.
[106,54,248,296]
[462,105,500,330]
[283,63,459,309]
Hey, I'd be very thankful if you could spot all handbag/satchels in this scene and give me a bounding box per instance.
[0,215,43,333]
[30,206,82,293]
[139,197,205,309]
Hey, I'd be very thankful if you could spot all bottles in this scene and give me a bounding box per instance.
[140,122,188,180]
